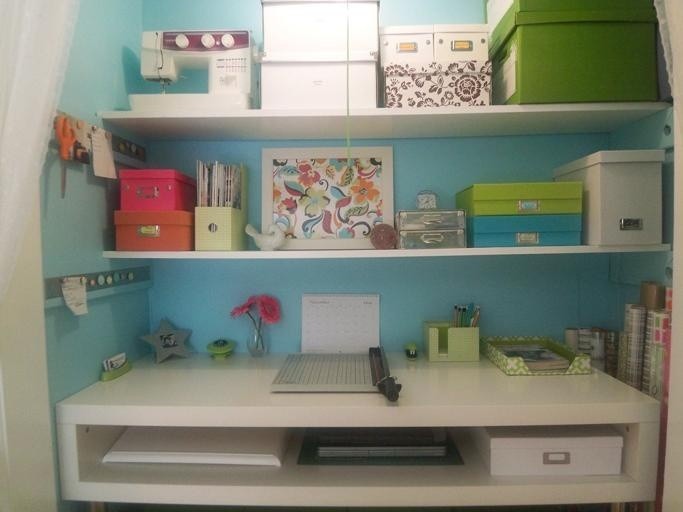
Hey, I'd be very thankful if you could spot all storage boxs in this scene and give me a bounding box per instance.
[112,208,195,253]
[487,0,662,106]
[118,167,200,213]
[378,22,492,66]
[469,424,624,478]
[256,2,383,111]
[463,215,587,247]
[550,146,667,249]
[383,60,495,108]
[454,180,585,216]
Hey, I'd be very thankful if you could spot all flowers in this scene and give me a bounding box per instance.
[226,290,287,329]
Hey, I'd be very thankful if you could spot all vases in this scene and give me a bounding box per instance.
[247,326,267,356]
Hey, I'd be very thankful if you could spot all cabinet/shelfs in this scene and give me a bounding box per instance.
[103,244,673,261]
[103,99,670,141]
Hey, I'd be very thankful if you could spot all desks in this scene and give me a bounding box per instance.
[53,351,664,507]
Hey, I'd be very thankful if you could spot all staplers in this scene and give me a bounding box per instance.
[404,342,416,360]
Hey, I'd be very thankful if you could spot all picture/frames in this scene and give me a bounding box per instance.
[261,147,395,250]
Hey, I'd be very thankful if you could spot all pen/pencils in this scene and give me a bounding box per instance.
[454,305,480,327]
